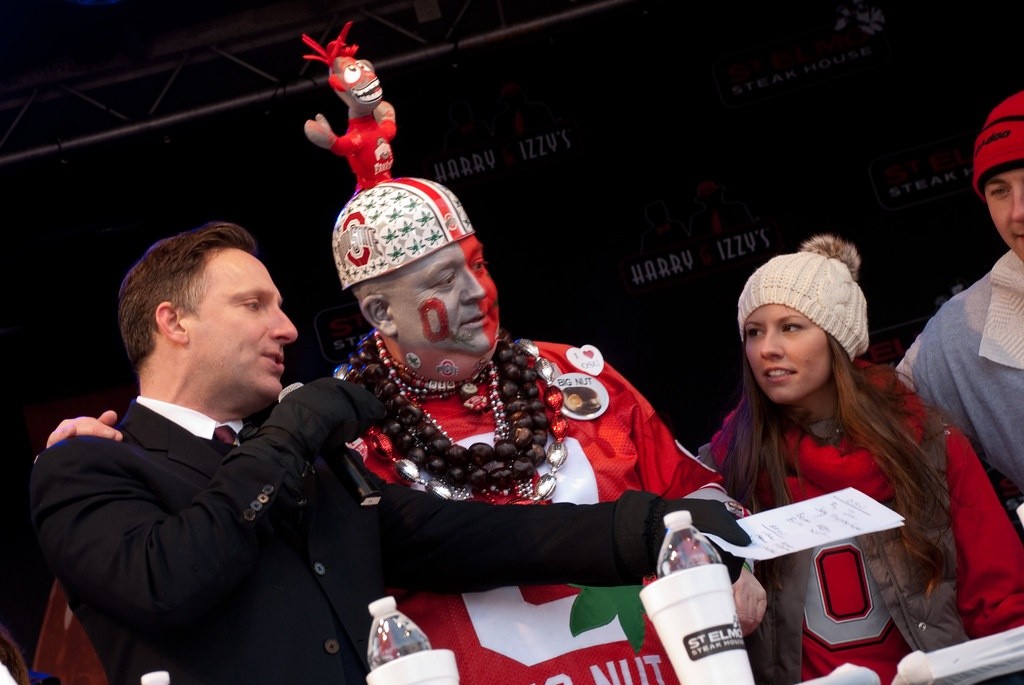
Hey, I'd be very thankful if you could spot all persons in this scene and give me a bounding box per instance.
[893,90,1024,496]
[30,222,752,684]
[46,178,767,685]
[697,235,1024,685]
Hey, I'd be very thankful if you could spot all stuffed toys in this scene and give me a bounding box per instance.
[302,22,396,187]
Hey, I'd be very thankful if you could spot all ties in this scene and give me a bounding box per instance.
[213,425,238,447]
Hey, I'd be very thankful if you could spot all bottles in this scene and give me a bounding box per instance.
[656,510,721,579]
[366,595,429,672]
[138,671,171,685]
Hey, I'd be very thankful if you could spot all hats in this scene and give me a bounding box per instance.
[302,21,476,291]
[973,89,1024,202]
[738,235,869,362]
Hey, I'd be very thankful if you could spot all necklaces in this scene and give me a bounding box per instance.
[331,325,568,506]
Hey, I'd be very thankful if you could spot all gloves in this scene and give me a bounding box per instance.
[613,489,751,584]
[220,378,386,524]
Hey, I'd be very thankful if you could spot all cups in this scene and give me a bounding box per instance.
[365,649,460,685]
[639,565,757,685]
